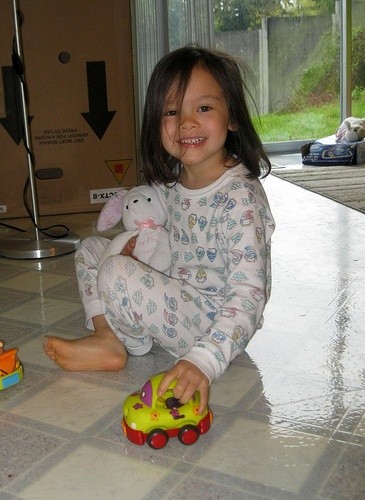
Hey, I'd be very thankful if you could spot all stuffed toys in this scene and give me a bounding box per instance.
[95,186,170,279]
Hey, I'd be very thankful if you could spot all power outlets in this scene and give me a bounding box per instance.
[0,205,6,213]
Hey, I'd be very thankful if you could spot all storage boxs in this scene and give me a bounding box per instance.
[301,135,365,165]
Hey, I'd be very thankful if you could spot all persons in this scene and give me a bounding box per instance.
[42,46,276,415]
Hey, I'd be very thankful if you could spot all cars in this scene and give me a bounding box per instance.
[122,372,213,449]
[0,340,24,391]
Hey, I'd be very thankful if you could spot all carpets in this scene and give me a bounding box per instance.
[270,162,365,214]
